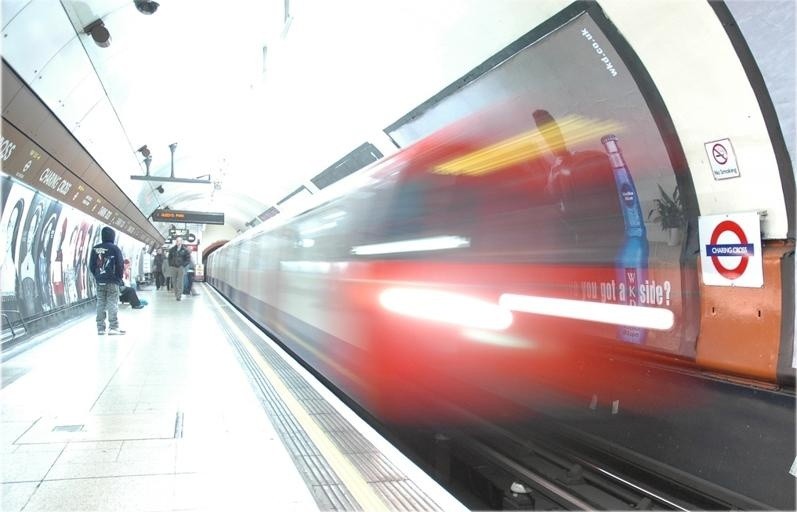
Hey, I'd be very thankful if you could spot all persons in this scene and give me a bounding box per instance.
[0,195,100,320]
[89,227,127,335]
[119,281,144,309]
[153,236,200,302]
[123,259,130,279]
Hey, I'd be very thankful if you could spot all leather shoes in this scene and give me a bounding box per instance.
[132,304,143,309]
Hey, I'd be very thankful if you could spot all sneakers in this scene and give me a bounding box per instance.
[98,330,106,335]
[108,328,125,335]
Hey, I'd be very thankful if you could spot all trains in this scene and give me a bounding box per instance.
[205,78,729,455]
